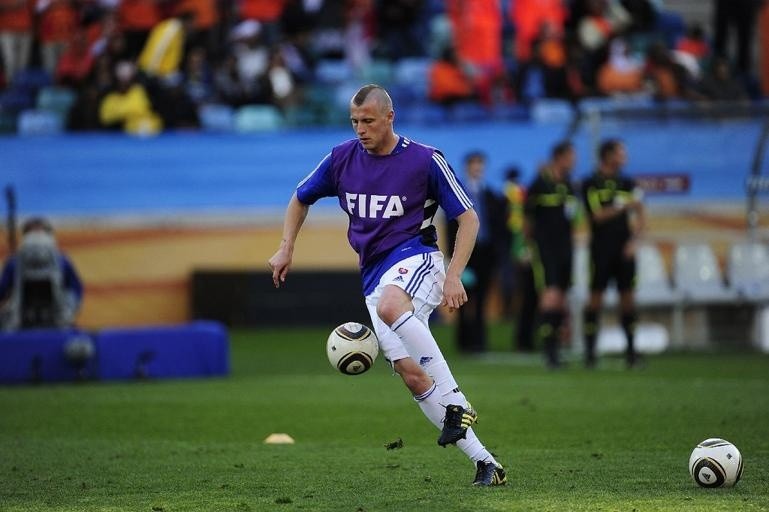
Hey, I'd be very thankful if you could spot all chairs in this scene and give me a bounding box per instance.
[566,234,767,346]
[4,3,766,131]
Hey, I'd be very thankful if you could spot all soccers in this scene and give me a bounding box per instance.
[327,323,379,375]
[688,438,744,489]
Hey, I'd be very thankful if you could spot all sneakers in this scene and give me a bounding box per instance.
[437,401,479,448]
[472,460,506,486]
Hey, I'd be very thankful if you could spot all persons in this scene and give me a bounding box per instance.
[522,139,579,370]
[2,212,85,327]
[498,164,544,349]
[445,138,510,353]
[578,138,650,368]
[271,83,512,490]
